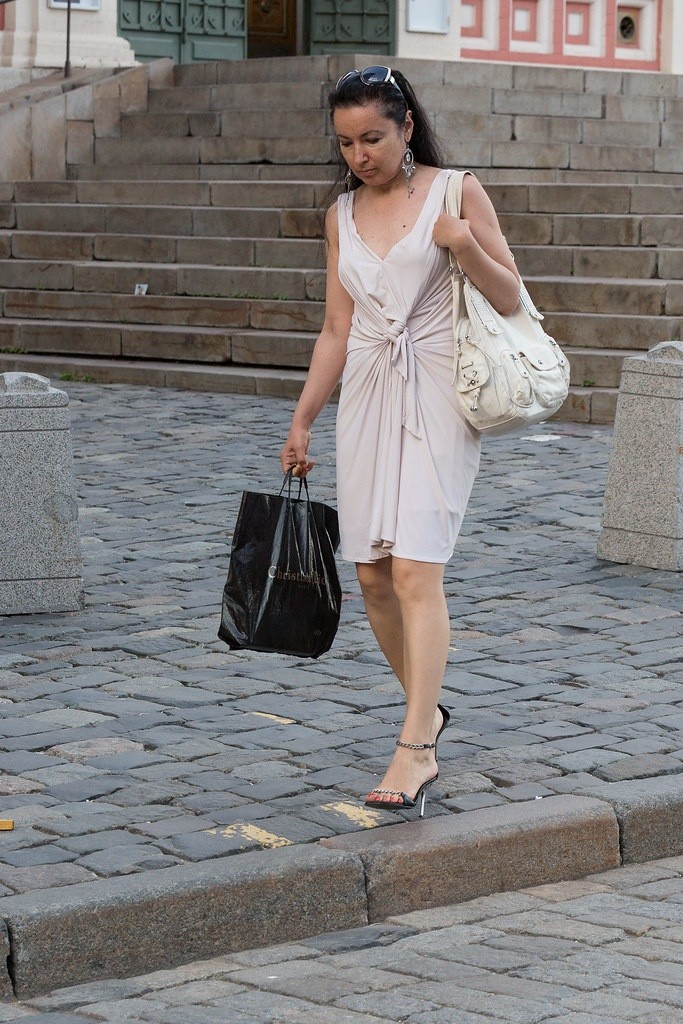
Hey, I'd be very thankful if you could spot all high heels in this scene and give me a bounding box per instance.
[431,703,448,763]
[364,769,438,817]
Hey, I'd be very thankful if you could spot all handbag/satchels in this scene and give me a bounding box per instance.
[443,170,574,438]
[214,464,342,661]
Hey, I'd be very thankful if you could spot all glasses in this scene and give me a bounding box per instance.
[334,65,411,110]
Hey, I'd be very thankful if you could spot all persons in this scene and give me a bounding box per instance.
[281,65,521,817]
